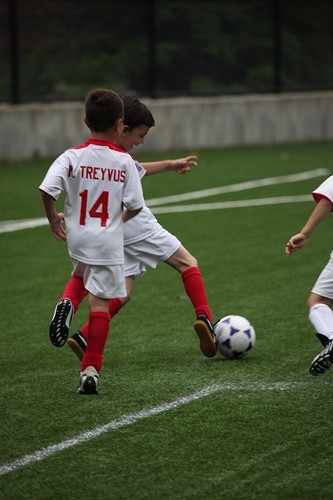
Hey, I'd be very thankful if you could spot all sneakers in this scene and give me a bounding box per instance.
[193,314,218,357]
[76,365,100,394]
[308,338,333,376]
[67,330,88,362]
[49,297,75,347]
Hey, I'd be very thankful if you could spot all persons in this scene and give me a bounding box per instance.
[38,89,145,395]
[285,174,333,376]
[68,95,217,361]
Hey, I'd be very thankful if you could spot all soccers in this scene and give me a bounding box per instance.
[212,314,256,358]
